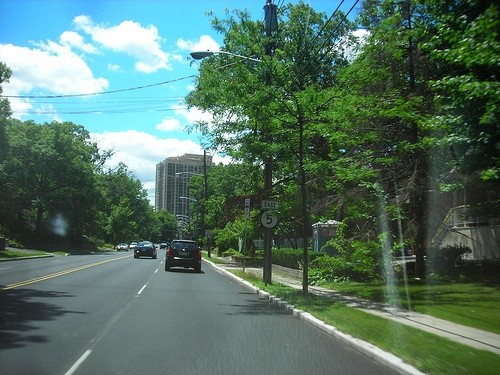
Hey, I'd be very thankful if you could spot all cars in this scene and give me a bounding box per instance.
[134,241,157,259]
[160,243,167,249]
[117,243,129,251]
[129,242,138,250]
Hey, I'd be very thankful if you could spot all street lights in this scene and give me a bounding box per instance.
[179,197,197,201]
[192,50,287,64]
[175,214,189,227]
[175,172,203,177]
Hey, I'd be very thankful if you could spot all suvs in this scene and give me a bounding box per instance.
[165,239,201,273]
